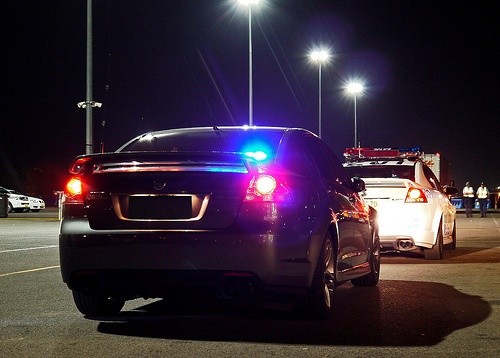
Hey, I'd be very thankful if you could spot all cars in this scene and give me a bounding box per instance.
[341,145,459,260]
[0,187,45,213]
[57,126,380,319]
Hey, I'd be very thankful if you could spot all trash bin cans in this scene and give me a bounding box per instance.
[0,194,10,218]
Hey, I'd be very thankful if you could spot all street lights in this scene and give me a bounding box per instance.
[309,46,333,138]
[344,79,364,147]
[237,0,261,125]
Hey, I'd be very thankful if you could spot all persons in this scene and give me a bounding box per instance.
[475,182,490,217]
[446,180,459,199]
[462,181,474,218]
